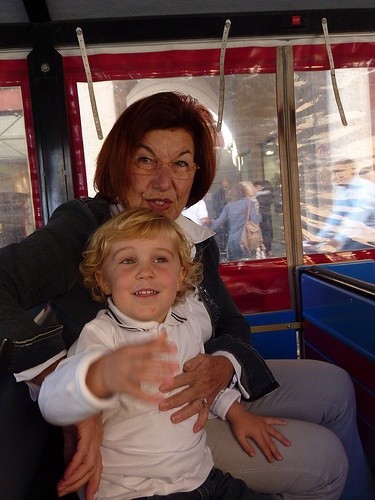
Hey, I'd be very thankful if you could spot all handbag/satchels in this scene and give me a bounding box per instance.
[240,221,264,255]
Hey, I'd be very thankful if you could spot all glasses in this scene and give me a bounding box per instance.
[132,155,198,180]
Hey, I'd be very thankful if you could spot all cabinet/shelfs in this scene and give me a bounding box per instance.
[295,259,375,470]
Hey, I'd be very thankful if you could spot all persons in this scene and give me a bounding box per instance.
[38,209,292,499]
[1,93,375,500]
[181,158,375,260]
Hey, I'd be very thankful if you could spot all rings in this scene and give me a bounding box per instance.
[199,397,208,409]
